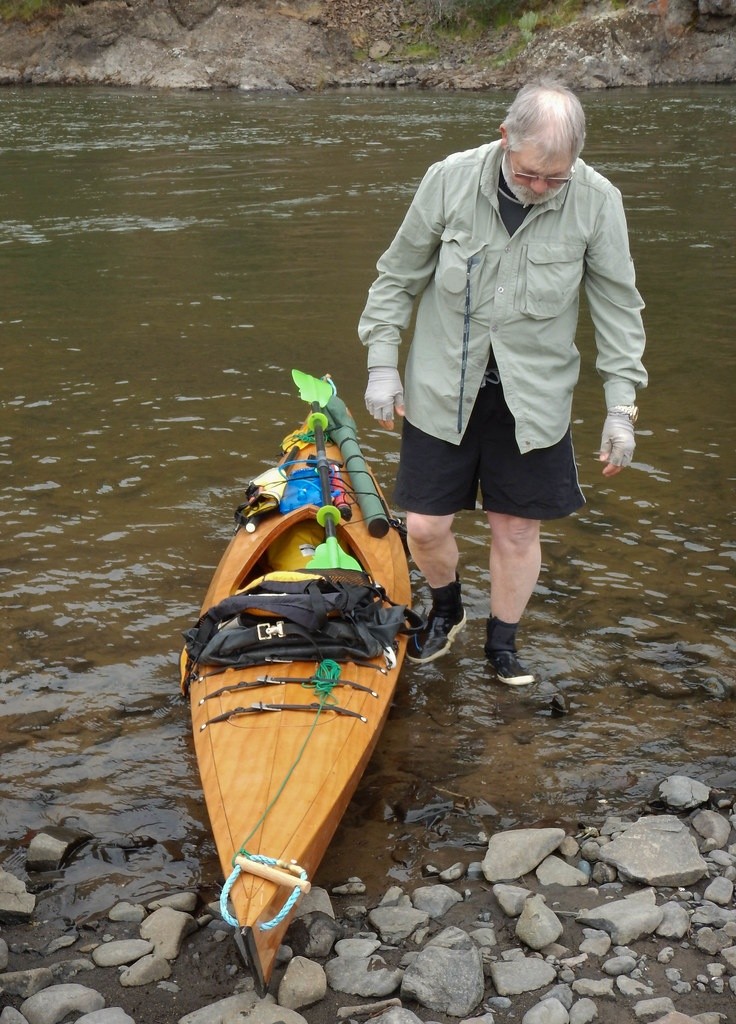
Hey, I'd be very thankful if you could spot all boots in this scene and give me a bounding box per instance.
[405,568,467,662]
[484,613,534,685]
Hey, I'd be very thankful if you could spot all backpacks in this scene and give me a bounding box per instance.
[181,565,425,668]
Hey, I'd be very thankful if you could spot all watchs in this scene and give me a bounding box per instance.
[608,405,639,423]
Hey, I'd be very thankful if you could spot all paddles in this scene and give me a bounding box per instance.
[289,367,361,571]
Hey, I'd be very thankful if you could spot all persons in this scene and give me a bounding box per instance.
[357,73,650,688]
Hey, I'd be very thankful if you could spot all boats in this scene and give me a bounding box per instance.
[175,372,416,998]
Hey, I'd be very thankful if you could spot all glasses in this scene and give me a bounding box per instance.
[510,147,574,189]
